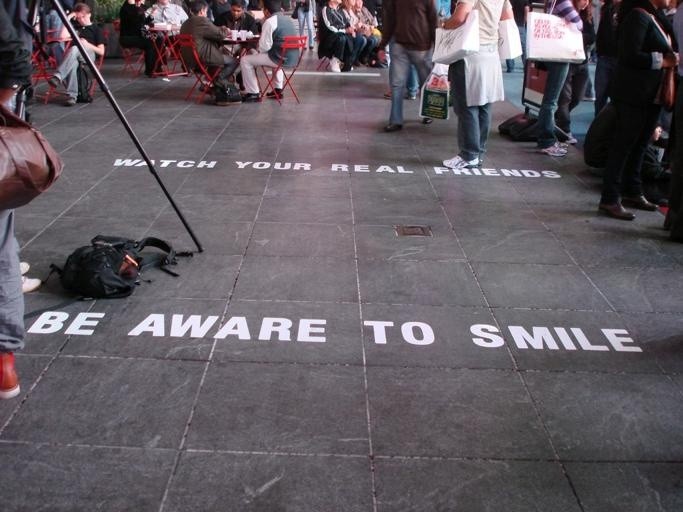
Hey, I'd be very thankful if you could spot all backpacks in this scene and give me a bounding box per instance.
[76,66,93,104]
[25,233,195,324]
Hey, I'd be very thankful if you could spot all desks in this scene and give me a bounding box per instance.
[218,36,263,97]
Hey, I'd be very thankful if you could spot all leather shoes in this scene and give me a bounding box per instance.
[21,276,42,295]
[0,350,20,400]
[19,261,30,276]
[342,56,388,72]
[598,202,636,221]
[384,122,402,132]
[200,81,283,106]
[620,195,659,212]
[422,118,434,124]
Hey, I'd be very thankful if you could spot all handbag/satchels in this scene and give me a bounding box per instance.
[498,19,524,61]
[0,103,65,211]
[431,2,482,65]
[525,0,587,64]
[663,66,674,112]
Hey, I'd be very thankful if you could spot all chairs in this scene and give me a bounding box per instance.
[172,34,234,104]
[312,19,331,70]
[24,21,187,104]
[256,35,307,106]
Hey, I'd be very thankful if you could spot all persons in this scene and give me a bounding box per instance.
[445,1,515,169]
[598,0,681,222]
[3,0,682,155]
[663,92,683,247]
[0,3,34,402]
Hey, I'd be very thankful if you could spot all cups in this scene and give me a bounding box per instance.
[230,30,246,41]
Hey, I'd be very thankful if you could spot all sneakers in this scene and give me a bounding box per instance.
[383,89,392,99]
[64,96,77,107]
[535,131,578,157]
[330,58,342,74]
[442,155,480,170]
[48,77,60,88]
